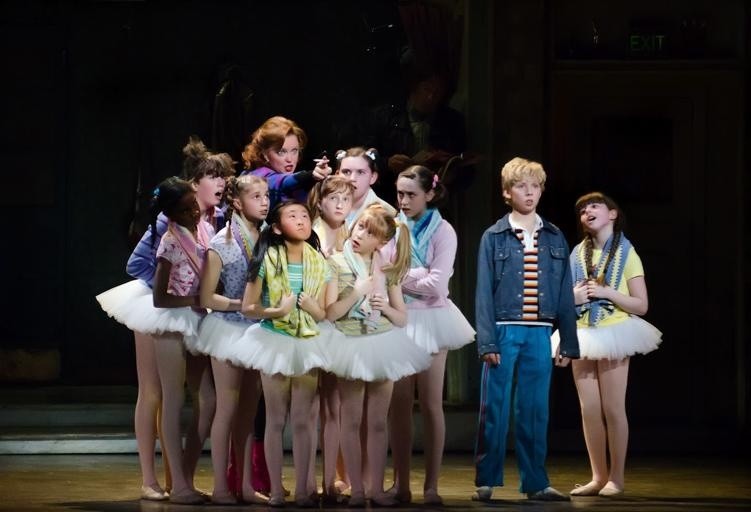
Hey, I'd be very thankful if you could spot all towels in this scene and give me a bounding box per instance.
[349,186,380,235]
[169,219,211,280]
[343,238,387,334]
[262,240,326,337]
[230,209,270,268]
[314,218,346,256]
[569,229,633,326]
[392,208,438,304]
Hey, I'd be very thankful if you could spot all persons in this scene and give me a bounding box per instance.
[93,115,479,508]
[549,189,665,501]
[364,61,484,223]
[473,155,581,503]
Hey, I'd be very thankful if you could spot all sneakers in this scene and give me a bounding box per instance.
[529,482,624,500]
[297,487,442,507]
[140,484,290,506]
[473,487,492,501]
